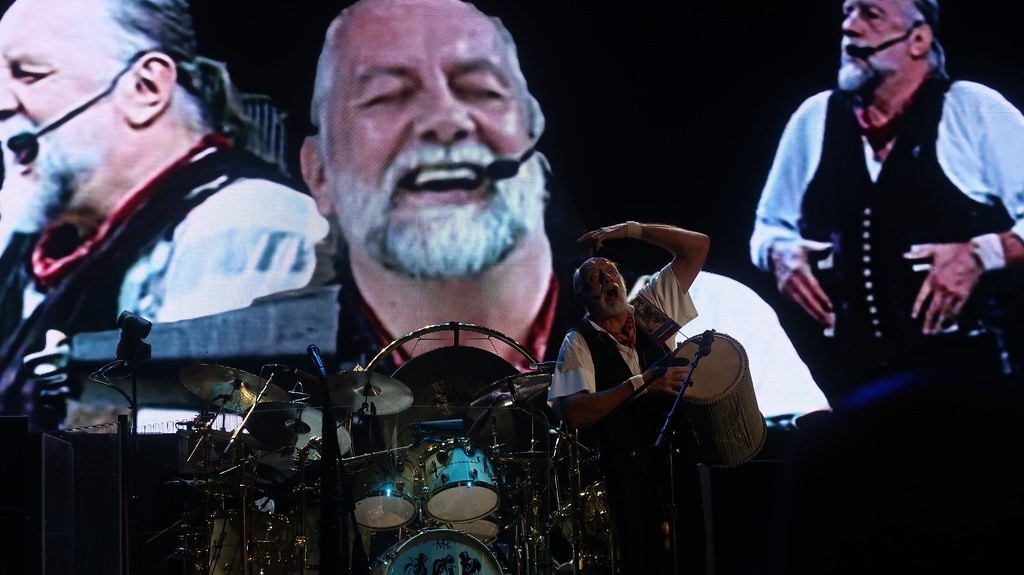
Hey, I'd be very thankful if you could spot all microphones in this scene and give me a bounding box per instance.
[409,419,465,433]
[854,21,922,58]
[307,344,328,385]
[586,294,600,299]
[265,363,293,373]
[7,50,149,152]
[696,330,714,356]
[177,422,196,427]
[487,146,535,182]
[466,395,503,440]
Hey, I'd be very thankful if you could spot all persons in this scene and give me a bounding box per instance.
[63,0,568,376]
[0,2,335,329]
[746,2,1024,339]
[538,216,713,471]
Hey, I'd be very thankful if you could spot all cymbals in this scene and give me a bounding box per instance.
[320,371,416,415]
[511,447,549,458]
[171,362,291,414]
[243,401,354,461]
[470,370,554,411]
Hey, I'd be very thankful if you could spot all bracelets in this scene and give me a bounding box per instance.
[627,219,643,239]
[971,234,1006,273]
[629,374,648,398]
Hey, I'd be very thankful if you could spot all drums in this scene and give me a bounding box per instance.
[563,449,616,538]
[374,527,504,575]
[667,330,769,468]
[181,492,250,575]
[420,434,502,524]
[347,453,419,532]
[253,479,361,574]
